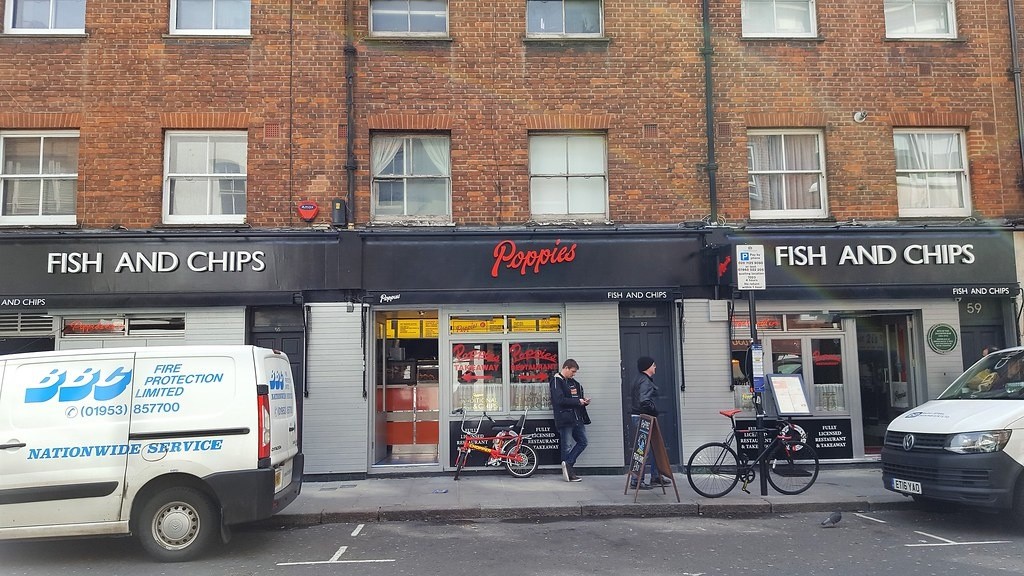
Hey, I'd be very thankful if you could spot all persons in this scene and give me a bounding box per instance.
[549,359,591,482]
[630,357,671,490]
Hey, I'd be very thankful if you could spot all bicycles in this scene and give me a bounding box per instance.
[452,405,539,481]
[687,410,819,498]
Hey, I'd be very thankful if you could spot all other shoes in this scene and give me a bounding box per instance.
[650,477,671,487]
[630,481,653,490]
[561,461,570,482]
[569,474,582,482]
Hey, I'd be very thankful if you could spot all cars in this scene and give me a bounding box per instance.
[880,344,1024,529]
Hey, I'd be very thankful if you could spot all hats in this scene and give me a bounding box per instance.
[637,357,654,372]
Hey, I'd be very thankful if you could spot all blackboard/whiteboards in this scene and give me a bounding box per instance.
[628,414,654,480]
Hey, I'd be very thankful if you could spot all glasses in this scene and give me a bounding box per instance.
[652,364,656,368]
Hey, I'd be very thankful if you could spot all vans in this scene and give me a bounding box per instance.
[0,344,305,562]
